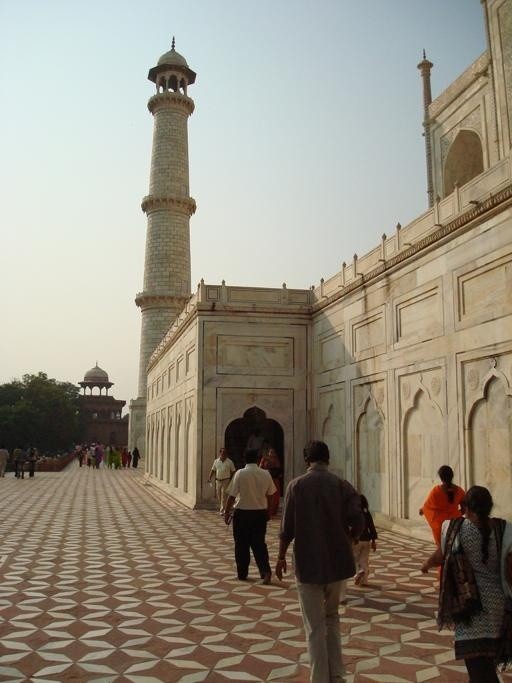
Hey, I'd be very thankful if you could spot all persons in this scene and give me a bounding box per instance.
[77,441,140,469]
[419,465,466,548]
[0,445,38,479]
[209,430,280,584]
[352,494,378,587]
[275,440,364,683]
[421,485,512,683]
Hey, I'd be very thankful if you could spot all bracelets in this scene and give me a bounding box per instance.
[277,555,285,561]
